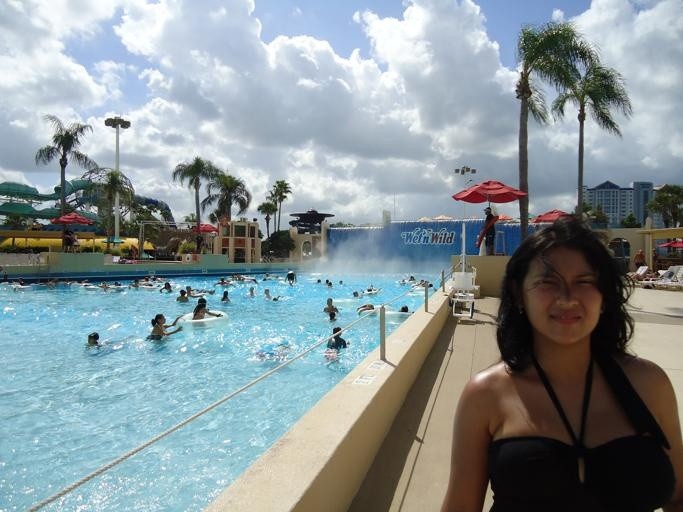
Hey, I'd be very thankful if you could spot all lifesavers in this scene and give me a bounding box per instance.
[80,278,185,294]
[412,283,434,294]
[359,304,413,322]
[177,310,229,331]
[216,276,252,287]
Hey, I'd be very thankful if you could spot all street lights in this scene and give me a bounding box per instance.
[452,165,477,220]
[103,115,132,248]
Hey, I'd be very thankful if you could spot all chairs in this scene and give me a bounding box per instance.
[622,261,682,292]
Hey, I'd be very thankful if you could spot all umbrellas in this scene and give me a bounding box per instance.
[193,224,219,233]
[658,241,683,248]
[531,209,577,223]
[451,180,528,207]
[50,211,92,224]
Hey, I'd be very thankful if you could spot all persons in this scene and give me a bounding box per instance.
[484,207,496,256]
[625,248,660,281]
[440,220,683,512]
